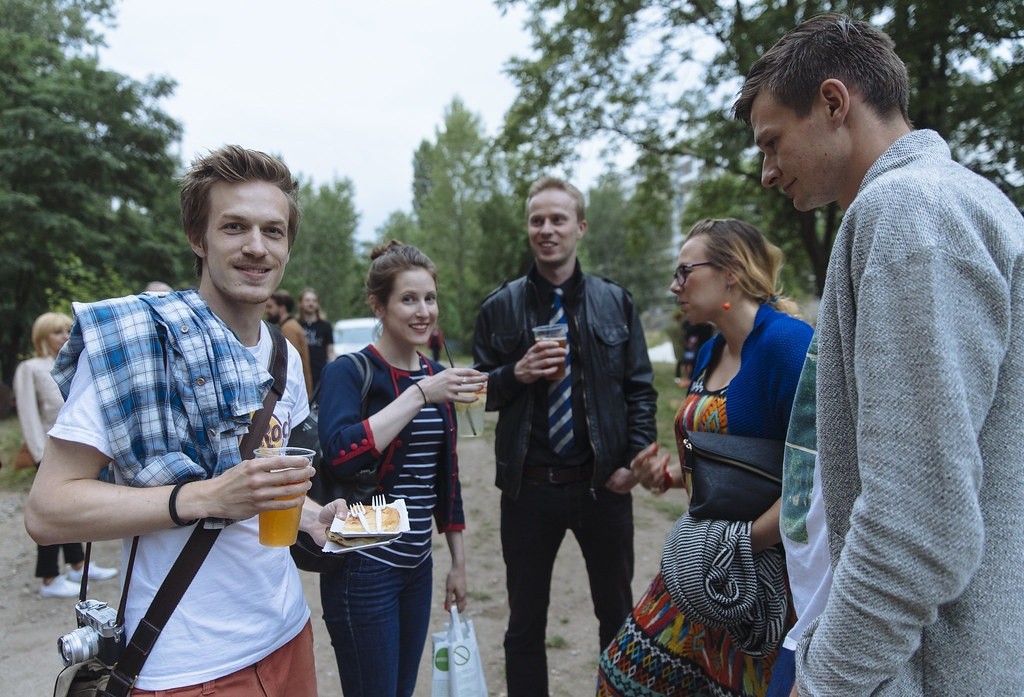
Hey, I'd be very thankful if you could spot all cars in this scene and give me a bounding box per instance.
[332,317,384,357]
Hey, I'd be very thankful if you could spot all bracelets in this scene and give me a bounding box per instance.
[169,476,199,527]
[665,471,673,492]
[414,382,427,406]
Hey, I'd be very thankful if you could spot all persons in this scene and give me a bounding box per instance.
[426,324,445,362]
[474,177,659,697]
[264,288,314,401]
[23,145,348,697]
[628,216,816,697]
[315,239,491,697]
[144,281,174,293]
[730,12,1023,697]
[13,312,119,597]
[296,286,335,389]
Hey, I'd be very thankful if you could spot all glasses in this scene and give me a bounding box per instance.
[673,257,721,285]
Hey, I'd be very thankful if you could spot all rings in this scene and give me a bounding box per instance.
[649,485,659,495]
[462,376,466,383]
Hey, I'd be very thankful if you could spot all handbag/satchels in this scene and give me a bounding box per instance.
[52,657,130,697]
[282,351,373,571]
[682,429,786,522]
[430,598,488,697]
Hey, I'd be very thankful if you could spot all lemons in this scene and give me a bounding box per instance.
[455,396,483,410]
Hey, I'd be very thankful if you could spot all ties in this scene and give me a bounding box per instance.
[547,288,574,454]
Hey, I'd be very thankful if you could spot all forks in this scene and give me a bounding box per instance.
[371,493,387,534]
[349,501,370,534]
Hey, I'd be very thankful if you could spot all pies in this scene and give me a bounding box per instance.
[325,527,386,546]
[344,504,399,532]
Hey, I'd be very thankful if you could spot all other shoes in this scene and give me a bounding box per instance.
[67,562,119,582]
[42,574,89,597]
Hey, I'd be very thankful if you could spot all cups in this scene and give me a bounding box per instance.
[531,325,567,379]
[454,372,489,438]
[253,447,316,546]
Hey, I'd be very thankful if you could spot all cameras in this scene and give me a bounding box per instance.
[57,598,126,668]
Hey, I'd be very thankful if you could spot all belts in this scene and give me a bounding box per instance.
[521,463,594,483]
